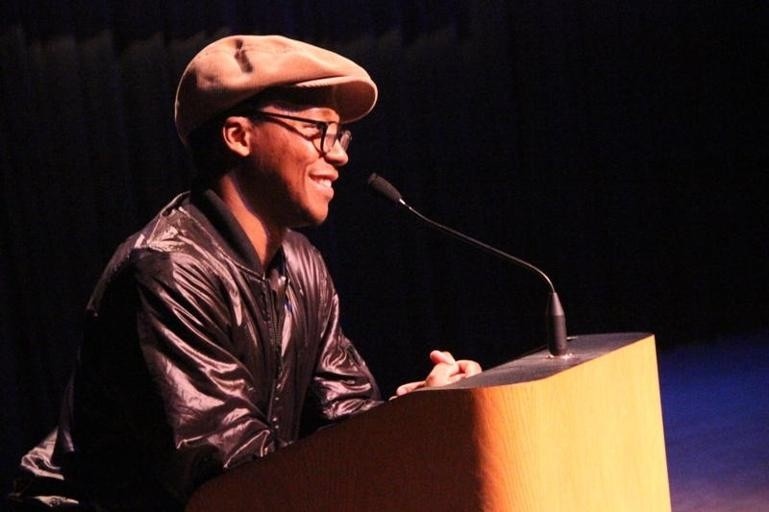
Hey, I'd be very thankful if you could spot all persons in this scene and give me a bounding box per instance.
[20,35,481,511]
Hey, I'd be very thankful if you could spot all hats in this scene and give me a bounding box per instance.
[174,35,379,144]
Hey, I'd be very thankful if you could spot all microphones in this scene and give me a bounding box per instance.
[366,172,569,357]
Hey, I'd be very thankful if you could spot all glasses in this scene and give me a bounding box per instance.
[248,111,353,155]
